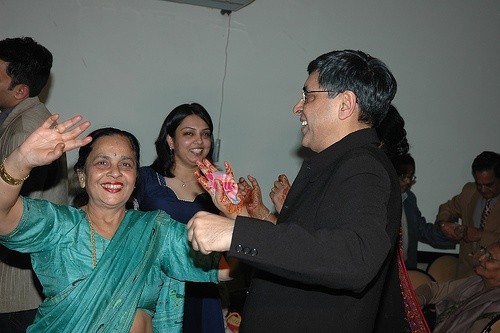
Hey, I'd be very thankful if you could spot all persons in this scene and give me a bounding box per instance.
[413,242,500,333]
[125,102,225,332]
[187,49,458,333]
[0,113,251,333]
[0,37,67,333]
[438,151,500,280]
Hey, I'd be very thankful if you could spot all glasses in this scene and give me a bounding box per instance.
[302,90,358,104]
[398,175,416,182]
[480,246,500,263]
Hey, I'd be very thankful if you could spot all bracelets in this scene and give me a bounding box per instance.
[262,211,272,220]
[0,158,29,186]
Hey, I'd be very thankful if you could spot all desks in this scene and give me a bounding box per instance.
[417,241,460,254]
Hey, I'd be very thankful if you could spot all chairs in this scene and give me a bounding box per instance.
[405,268,435,290]
[425,255,459,282]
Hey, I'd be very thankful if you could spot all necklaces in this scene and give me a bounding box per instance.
[175,176,196,187]
[85,212,97,268]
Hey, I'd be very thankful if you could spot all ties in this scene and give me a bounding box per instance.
[480,199,491,232]
